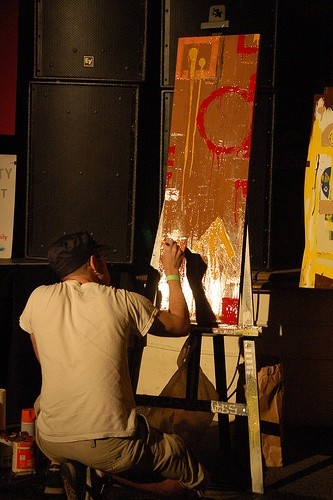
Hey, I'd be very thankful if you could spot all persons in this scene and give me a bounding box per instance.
[17,240,216,500]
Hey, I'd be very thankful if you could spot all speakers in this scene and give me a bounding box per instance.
[156,0,278,270]
[24,0,151,265]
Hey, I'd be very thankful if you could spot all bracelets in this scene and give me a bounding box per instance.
[165,274,181,283]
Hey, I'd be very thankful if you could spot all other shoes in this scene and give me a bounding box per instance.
[61,462,87,498]
[43,467,65,494]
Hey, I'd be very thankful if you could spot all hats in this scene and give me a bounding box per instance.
[49,230,110,278]
